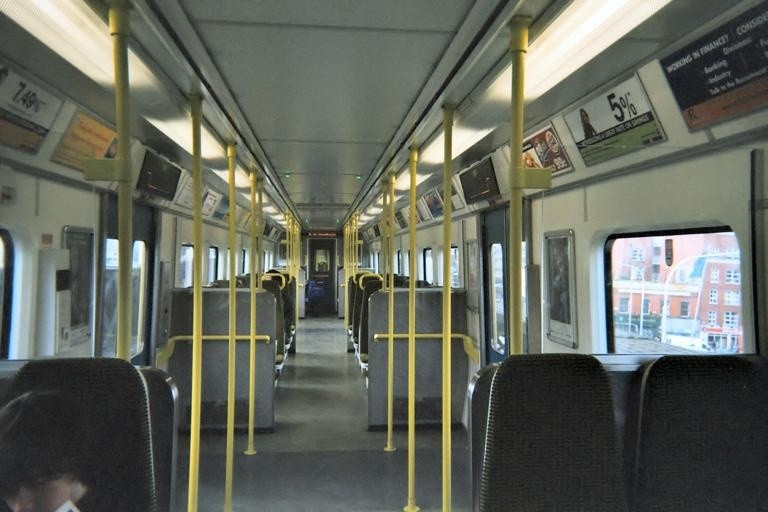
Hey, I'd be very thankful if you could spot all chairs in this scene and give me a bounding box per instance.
[169,262,307,433]
[344,273,466,431]
[630,356,768,511]
[475,354,628,512]
[5,355,158,512]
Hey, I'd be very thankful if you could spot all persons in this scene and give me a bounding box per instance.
[1,390,113,512]
[578,108,597,140]
